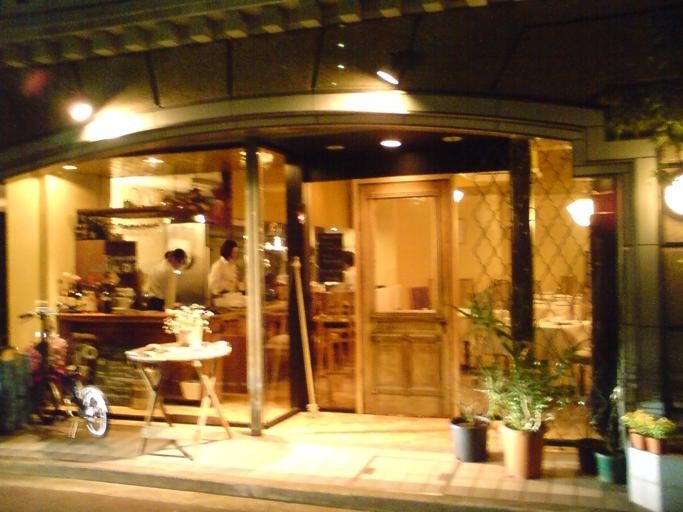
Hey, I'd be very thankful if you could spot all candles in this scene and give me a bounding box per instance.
[444,281,593,479]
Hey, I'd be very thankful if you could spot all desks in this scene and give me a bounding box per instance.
[125,342,233,460]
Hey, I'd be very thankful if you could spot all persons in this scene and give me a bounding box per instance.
[206,238,241,305]
[145,248,188,312]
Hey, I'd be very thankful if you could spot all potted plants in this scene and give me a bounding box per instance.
[586,332,682,512]
[162,304,214,346]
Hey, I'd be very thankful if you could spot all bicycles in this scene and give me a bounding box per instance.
[17,303,109,439]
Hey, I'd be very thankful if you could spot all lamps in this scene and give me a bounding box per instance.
[377,14,435,91]
